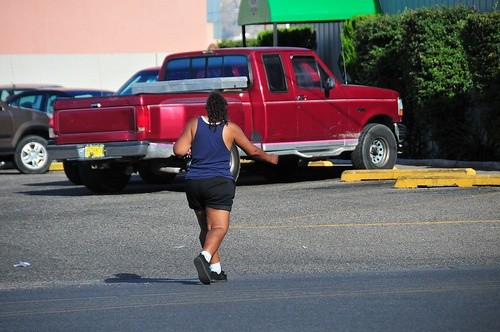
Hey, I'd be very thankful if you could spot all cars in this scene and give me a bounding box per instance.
[8,90,115,118]
[1,86,59,107]
[115,68,166,99]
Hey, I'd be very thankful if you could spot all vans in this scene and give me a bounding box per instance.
[0,98,50,176]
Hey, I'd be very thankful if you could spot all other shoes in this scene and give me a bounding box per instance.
[210,269,228,283]
[194,253,211,285]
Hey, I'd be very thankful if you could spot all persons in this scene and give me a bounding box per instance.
[173,92,279,285]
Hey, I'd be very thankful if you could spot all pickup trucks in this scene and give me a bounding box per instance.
[45,47,403,194]
